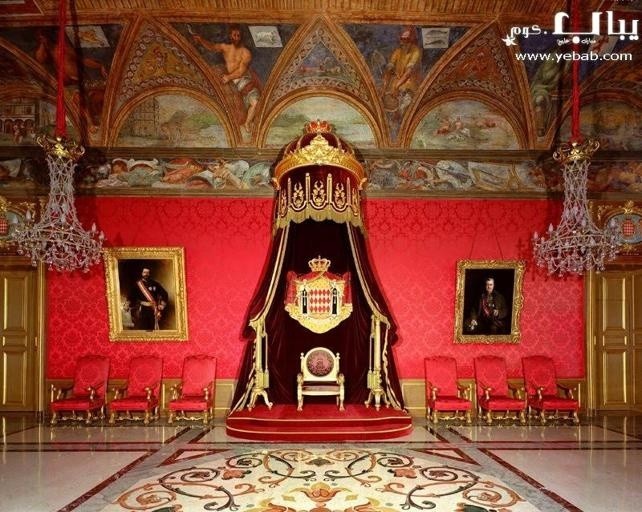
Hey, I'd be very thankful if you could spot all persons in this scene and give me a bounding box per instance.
[328,279,345,317]
[296,278,311,317]
[123,267,169,330]
[465,276,507,334]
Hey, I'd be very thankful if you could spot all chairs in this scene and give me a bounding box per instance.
[423,356,579,426]
[296,346,345,411]
[49,354,217,424]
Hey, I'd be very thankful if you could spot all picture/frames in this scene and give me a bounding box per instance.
[453,259,526,343]
[101,246,190,343]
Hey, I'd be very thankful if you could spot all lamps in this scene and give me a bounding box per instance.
[10,1,108,276]
[530,1,624,278]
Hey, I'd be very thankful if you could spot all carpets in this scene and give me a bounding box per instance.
[102,447,538,512]
